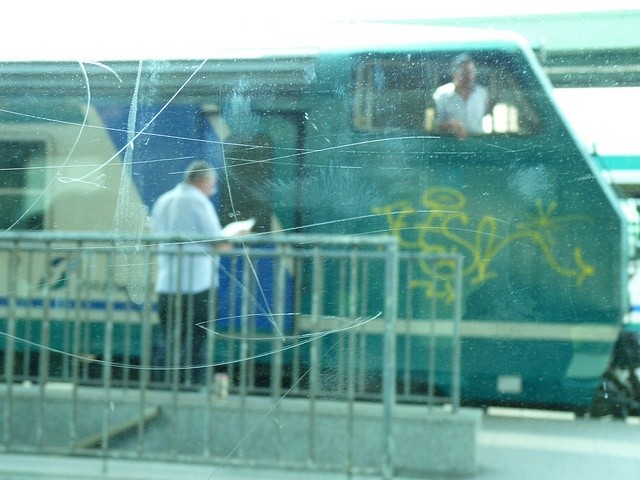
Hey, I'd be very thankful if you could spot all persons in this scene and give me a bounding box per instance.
[430,52,507,136]
[149,161,249,387]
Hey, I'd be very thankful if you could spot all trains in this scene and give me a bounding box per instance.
[1,46,640,423]
